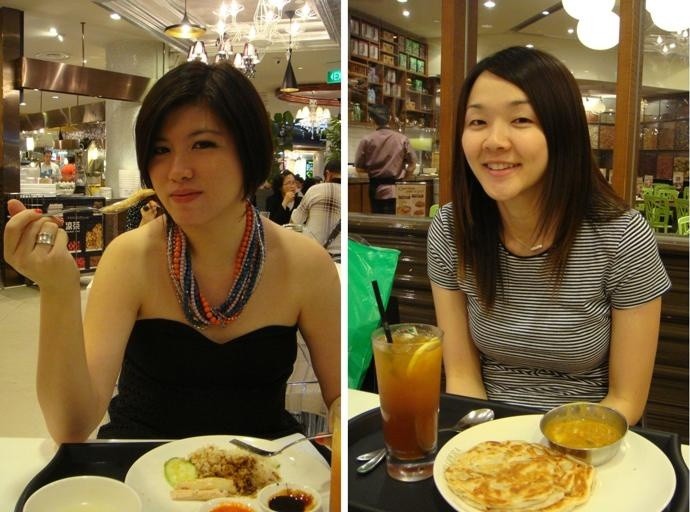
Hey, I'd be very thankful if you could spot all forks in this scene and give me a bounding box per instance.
[230,433,332,456]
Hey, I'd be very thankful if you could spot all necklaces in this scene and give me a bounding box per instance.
[508,216,558,251]
[162,202,264,329]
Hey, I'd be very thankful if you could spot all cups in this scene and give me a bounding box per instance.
[372,323,444,485]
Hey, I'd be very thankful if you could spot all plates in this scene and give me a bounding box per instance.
[125,435,330,512]
[429,415,677,512]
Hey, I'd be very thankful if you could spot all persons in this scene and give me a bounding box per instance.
[426,45,672,427]
[355,104,416,215]
[61,154,77,181]
[125,195,163,233]
[266,156,341,264]
[1,63,341,447]
[37,150,62,179]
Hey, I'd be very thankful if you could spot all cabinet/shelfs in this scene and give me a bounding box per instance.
[348,6,441,131]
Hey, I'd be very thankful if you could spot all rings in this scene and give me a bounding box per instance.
[34,232,55,247]
[143,204,149,211]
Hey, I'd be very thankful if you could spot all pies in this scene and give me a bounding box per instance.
[544,450,594,512]
[445,440,576,511]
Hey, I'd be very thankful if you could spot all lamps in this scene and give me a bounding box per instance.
[562,0,690,51]
[296,91,331,139]
[163,0,316,92]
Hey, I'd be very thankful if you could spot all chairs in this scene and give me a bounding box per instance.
[634,183,689,236]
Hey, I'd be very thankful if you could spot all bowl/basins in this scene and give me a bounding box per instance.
[541,402,627,466]
[14,474,141,512]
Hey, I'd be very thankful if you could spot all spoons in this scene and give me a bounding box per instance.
[358,404,494,477]
[8,202,128,219]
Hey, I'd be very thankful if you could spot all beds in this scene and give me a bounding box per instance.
[0,437,332,512]
